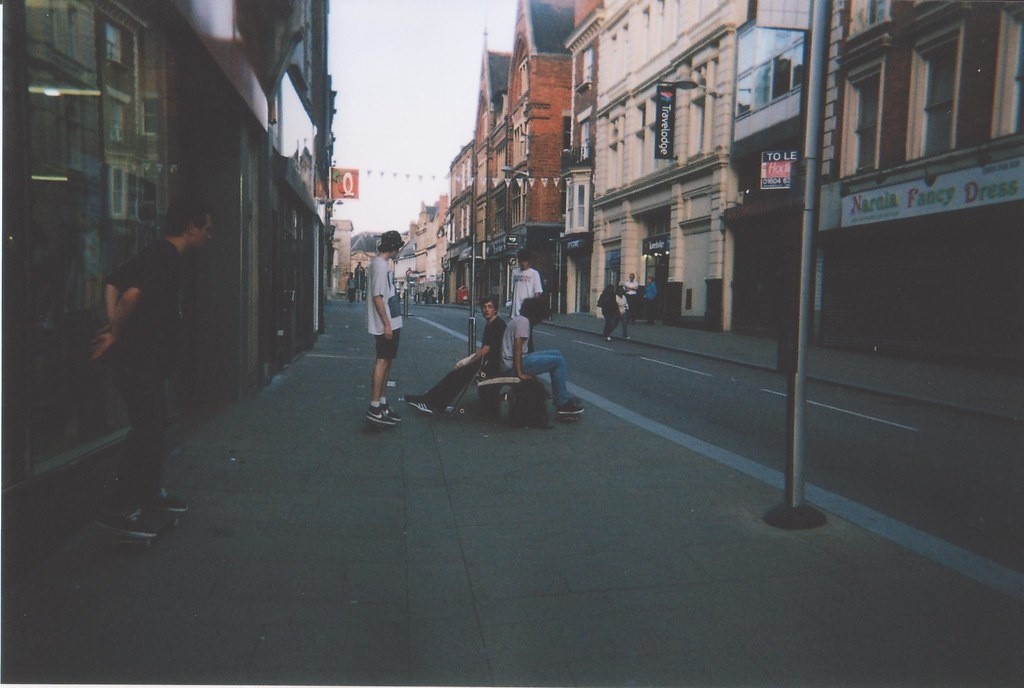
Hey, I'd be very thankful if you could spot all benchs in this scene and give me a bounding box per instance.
[476,377,522,426]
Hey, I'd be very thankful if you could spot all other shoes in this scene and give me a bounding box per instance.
[604,336,611,341]
[625,336,631,339]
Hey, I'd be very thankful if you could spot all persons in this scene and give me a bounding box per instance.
[510,249,543,353]
[86,200,215,538]
[345,272,443,305]
[595,273,656,341]
[406,296,507,417]
[501,298,585,414]
[366,230,406,425]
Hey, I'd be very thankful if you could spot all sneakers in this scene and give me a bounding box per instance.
[365,406,397,424]
[556,404,585,414]
[408,402,433,414]
[134,483,189,511]
[380,403,403,421]
[92,504,162,537]
[404,394,427,402]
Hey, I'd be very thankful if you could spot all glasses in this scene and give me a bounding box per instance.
[630,277,634,278]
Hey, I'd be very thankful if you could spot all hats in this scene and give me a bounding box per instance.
[378,230,405,252]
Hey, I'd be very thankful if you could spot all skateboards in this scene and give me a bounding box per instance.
[364,417,387,434]
[92,503,186,552]
[446,353,486,418]
[557,409,584,423]
